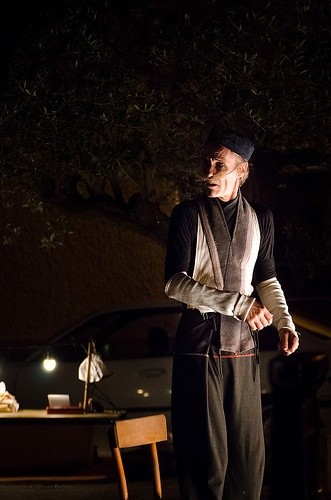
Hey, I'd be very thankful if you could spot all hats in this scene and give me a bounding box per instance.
[208,126,255,163]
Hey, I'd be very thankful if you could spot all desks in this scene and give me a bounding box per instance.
[0,410,167,500]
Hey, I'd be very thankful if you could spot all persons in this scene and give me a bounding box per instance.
[165,128,300,500]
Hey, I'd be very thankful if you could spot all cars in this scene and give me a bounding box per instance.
[0,301,331,474]
